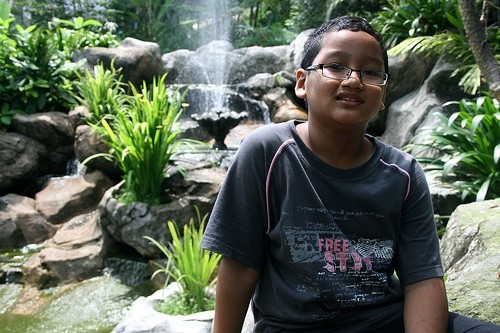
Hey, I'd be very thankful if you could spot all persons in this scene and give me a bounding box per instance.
[202,15,500,333]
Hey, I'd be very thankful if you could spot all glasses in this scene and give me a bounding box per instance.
[305,62,390,86]
